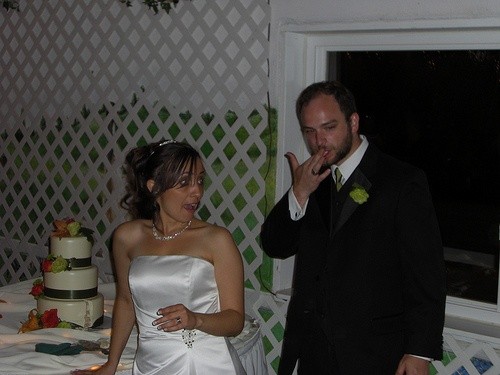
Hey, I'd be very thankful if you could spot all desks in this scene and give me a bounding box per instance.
[0,277,268,375]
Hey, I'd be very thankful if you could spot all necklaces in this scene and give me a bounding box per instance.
[150,219,192,240]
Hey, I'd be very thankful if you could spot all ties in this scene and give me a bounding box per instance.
[334,166,343,192]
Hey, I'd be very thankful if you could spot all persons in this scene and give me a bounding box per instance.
[260,80,449,375]
[69,139,246,375]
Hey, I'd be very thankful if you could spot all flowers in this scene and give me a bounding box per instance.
[39,255,72,273]
[28,279,44,300]
[40,308,72,330]
[349,188,369,204]
[50,216,96,247]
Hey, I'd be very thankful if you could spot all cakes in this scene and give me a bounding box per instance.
[32,219,105,328]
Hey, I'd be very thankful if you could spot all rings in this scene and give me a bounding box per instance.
[176,318,181,325]
[311,168,318,176]
